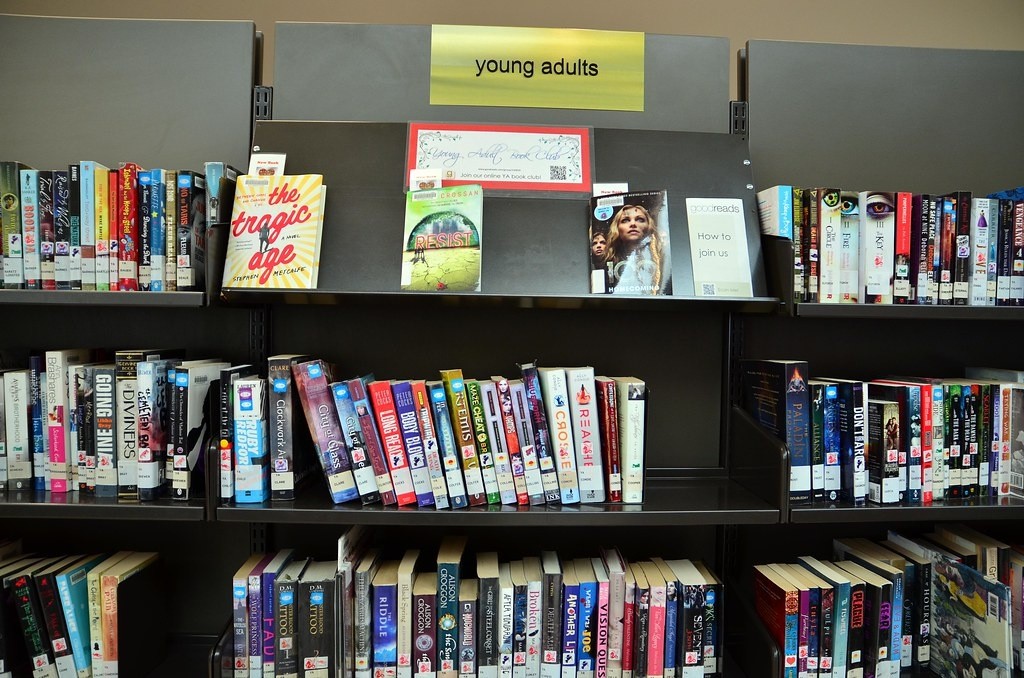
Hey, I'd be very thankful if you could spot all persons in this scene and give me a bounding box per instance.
[607,203,673,297]
[590,232,622,295]
[4,195,16,211]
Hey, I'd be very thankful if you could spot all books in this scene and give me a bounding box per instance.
[0,342,650,504]
[0,156,251,290]
[221,173,327,290]
[402,184,483,293]
[0,524,1021,677]
[732,352,1023,505]
[588,183,1024,307]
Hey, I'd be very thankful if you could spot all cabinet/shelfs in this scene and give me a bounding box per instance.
[0,18,234,678]
[220,20,736,677]
[730,39,1024,678]
